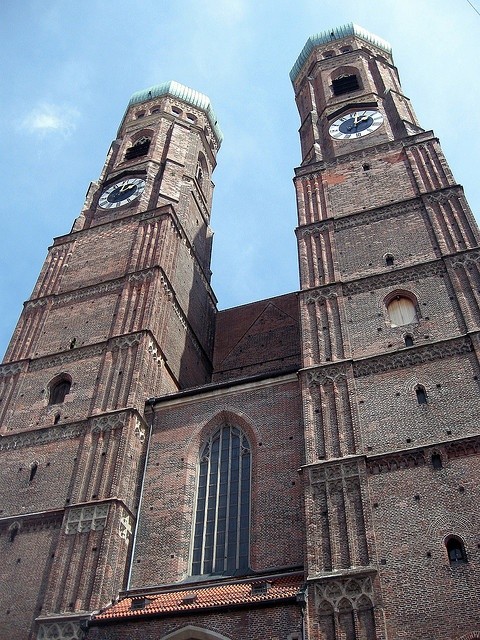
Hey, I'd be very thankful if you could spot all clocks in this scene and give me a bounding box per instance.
[98,178,146,210]
[328,110,384,140]
